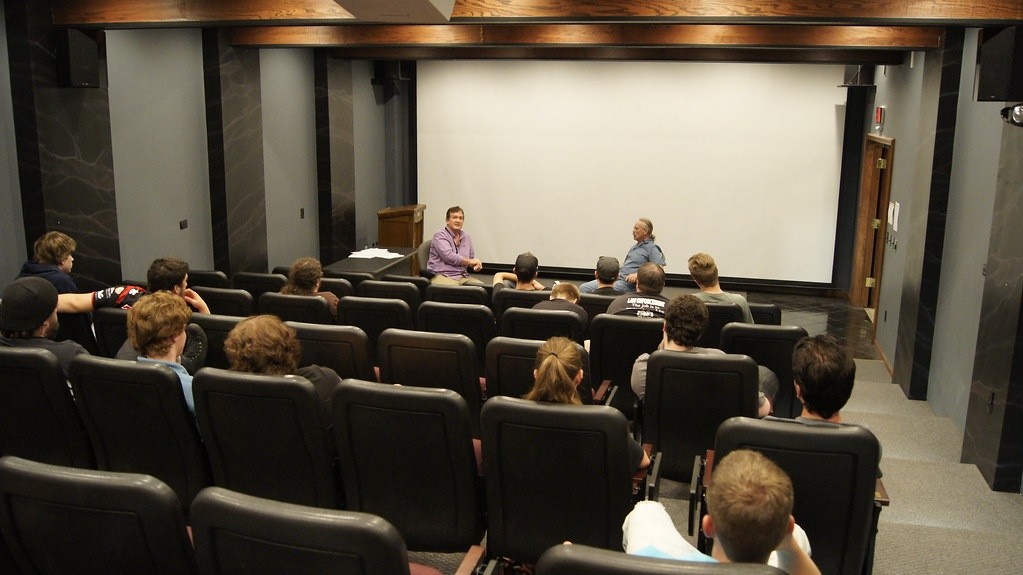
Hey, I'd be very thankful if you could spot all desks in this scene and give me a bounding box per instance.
[324,245,419,277]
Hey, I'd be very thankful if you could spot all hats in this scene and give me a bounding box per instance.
[1,276,59,339]
[514,252,538,275]
[597,256,620,280]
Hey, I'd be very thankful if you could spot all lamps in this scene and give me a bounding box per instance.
[999,103,1023,126]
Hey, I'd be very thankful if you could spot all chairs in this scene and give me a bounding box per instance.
[0,266,894,575]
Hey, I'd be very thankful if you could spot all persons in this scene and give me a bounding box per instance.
[688,253,755,324]
[579,218,666,293]
[525,335,649,472]
[56,258,211,313]
[426,206,485,286]
[792,334,882,480]
[622,450,822,575]
[591,256,625,295]
[492,252,551,291]
[606,262,670,318]
[631,294,777,418]
[16,232,97,354]
[0,277,90,400]
[532,283,587,329]
[127,291,195,413]
[225,315,342,457]
[281,258,340,315]
